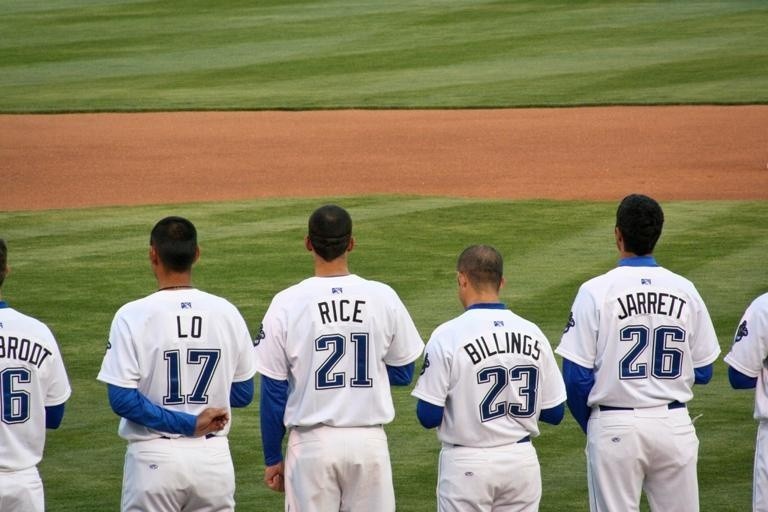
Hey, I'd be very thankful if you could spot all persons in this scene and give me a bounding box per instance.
[720,290,768,512]
[92,213,256,512]
[252,203,425,512]
[0,235,72,512]
[409,244,569,512]
[554,192,721,512]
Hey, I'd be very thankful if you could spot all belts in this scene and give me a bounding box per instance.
[599,400,685,410]
